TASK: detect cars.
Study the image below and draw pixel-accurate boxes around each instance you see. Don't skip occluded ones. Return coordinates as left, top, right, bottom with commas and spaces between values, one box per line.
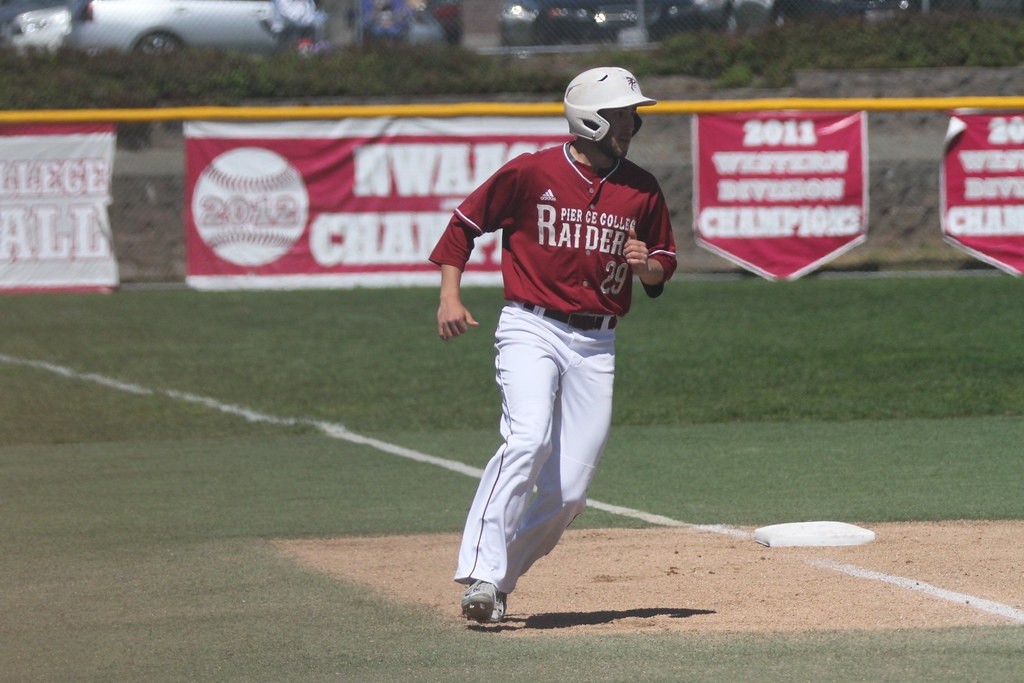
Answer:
11, 0, 319, 61
500, 0, 636, 45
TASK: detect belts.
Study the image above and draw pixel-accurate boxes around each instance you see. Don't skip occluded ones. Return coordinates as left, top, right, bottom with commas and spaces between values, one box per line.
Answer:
523, 302, 617, 331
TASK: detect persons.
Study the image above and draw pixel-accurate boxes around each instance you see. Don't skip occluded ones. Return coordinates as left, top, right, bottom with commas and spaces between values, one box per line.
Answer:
427, 66, 677, 623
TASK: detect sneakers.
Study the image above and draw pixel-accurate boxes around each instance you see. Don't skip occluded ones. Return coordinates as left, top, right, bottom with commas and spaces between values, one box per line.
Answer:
461, 580, 508, 625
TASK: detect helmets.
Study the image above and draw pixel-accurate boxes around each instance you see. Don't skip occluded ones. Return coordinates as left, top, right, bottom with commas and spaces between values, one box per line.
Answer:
564, 66, 659, 142
